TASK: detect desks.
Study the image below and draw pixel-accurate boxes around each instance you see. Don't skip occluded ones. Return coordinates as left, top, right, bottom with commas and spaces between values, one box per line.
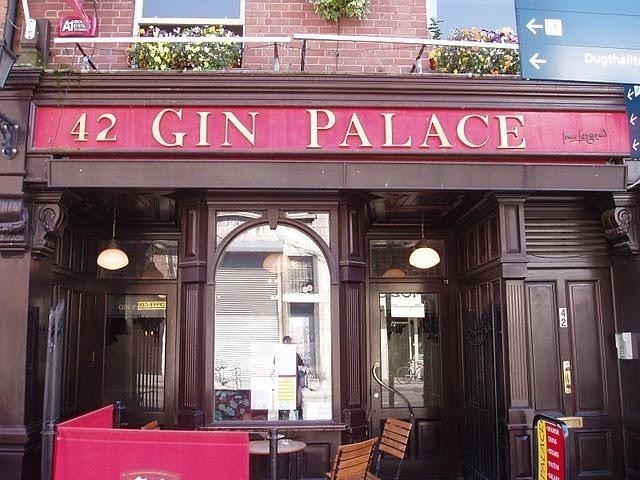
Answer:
249, 439, 307, 479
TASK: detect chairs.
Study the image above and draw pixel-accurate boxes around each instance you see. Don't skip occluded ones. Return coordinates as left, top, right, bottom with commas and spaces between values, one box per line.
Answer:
141, 420, 161, 430
296, 416, 413, 480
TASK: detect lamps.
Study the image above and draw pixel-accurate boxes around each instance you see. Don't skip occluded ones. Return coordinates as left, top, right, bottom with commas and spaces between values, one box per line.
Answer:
409, 208, 441, 269
0, 37, 20, 159
97, 201, 130, 270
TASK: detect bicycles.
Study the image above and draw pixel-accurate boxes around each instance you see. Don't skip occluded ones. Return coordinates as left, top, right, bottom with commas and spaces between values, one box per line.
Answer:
301, 352, 320, 391
397, 356, 425, 384
215, 366, 242, 390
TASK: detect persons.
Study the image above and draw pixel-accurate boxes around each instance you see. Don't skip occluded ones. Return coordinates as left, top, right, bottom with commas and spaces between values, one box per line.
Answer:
272, 335, 307, 421
